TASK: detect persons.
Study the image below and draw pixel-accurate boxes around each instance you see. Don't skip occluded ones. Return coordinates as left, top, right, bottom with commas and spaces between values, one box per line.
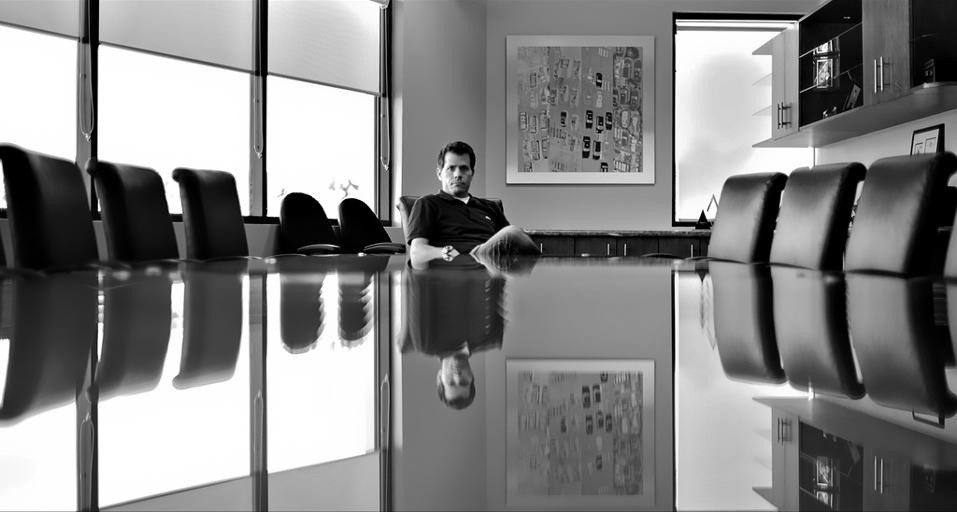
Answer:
401, 255, 508, 410
406, 137, 550, 258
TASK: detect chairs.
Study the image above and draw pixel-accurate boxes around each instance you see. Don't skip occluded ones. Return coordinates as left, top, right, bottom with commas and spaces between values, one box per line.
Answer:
275, 192, 505, 255
0, 144, 250, 271
0, 260, 242, 427
709, 152, 956, 278
710, 260, 954, 419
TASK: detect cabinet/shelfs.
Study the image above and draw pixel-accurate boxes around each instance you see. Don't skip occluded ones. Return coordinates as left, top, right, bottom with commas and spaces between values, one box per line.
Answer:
575, 236, 658, 257
527, 236, 574, 259
660, 236, 711, 259
750, 0, 956, 150
753, 395, 956, 511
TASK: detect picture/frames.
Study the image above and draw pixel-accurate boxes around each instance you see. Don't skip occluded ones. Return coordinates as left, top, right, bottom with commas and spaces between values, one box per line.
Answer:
504, 34, 657, 188
910, 123, 945, 155
502, 355, 656, 508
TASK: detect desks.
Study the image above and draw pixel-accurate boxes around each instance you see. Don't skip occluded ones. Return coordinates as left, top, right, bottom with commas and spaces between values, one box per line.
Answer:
3, 257, 956, 512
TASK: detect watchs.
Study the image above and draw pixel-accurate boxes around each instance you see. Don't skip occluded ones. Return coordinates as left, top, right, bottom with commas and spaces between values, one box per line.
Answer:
442, 245, 454, 256
443, 255, 453, 262
